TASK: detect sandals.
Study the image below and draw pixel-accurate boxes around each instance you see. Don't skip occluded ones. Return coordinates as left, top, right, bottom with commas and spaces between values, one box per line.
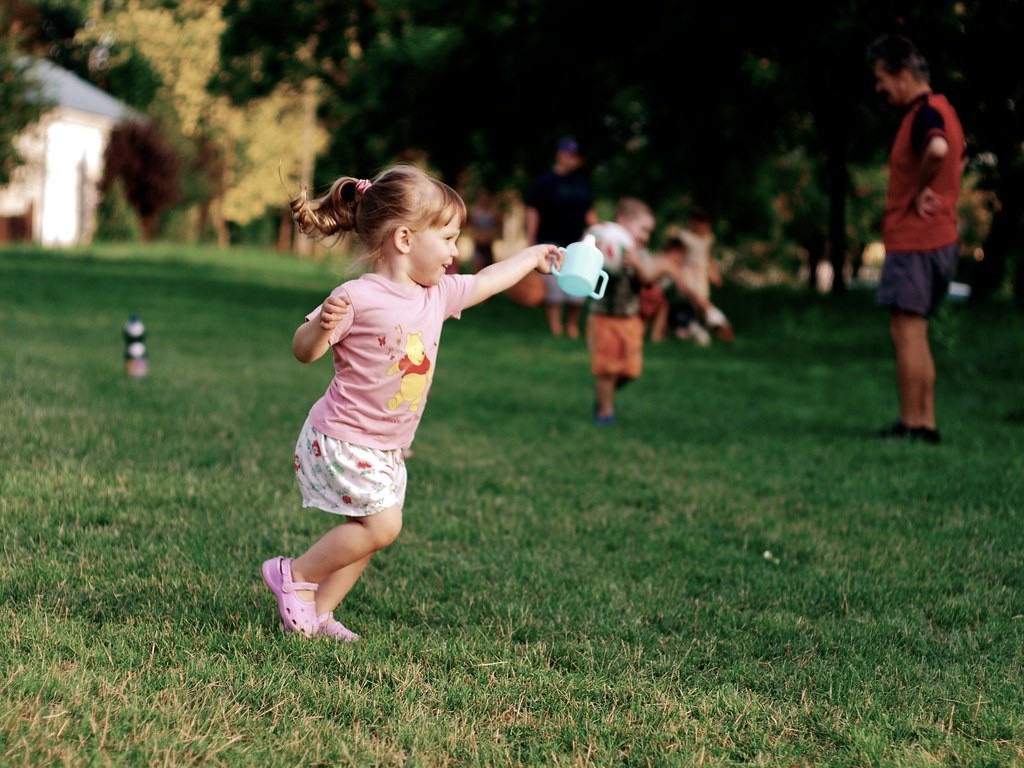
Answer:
263, 556, 320, 640
316, 611, 365, 644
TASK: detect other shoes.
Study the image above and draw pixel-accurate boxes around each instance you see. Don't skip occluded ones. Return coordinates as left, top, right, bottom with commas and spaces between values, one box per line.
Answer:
879, 420, 923, 440
918, 425, 942, 444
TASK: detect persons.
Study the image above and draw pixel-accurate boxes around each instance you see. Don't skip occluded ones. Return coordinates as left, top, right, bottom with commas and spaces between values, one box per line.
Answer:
456, 174, 738, 355
582, 197, 666, 425
257, 159, 565, 643
869, 38, 966, 447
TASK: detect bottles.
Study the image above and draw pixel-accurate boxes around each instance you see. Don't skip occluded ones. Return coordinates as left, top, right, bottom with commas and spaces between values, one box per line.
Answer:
122, 314, 149, 377
548, 233, 612, 301
